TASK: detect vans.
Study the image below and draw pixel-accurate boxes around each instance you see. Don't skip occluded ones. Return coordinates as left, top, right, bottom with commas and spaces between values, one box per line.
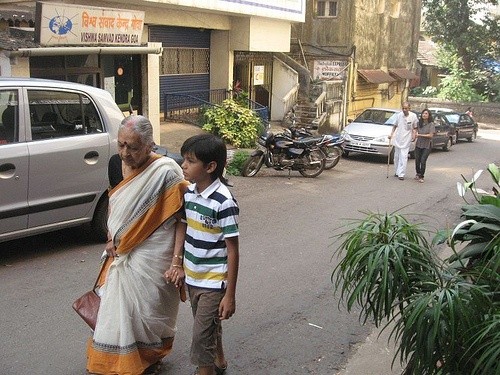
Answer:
0, 78, 126, 241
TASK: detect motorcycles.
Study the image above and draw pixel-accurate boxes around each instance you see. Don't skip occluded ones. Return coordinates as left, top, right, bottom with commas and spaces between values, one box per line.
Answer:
241, 120, 326, 179
284, 108, 346, 170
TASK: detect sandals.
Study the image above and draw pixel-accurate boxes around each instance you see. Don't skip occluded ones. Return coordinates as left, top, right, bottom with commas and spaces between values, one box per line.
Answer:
213, 361, 228, 375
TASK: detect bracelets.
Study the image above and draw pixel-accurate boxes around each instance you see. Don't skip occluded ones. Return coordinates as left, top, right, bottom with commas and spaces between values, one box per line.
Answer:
173, 253, 183, 260
169, 264, 184, 270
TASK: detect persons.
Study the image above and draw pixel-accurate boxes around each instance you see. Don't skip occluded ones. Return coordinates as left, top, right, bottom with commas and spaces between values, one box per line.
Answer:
165, 133, 240, 375
86, 115, 193, 375
414, 108, 435, 183
465, 107, 473, 118
387, 101, 419, 181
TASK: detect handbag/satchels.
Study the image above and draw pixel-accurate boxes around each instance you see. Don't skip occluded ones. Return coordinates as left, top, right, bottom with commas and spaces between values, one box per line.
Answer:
71, 289, 100, 331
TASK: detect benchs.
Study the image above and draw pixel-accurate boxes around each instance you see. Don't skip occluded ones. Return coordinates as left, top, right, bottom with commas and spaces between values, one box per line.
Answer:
37, 111, 96, 139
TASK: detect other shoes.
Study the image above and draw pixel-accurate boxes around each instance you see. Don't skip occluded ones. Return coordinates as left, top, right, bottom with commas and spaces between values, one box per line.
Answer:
415, 175, 424, 182
395, 173, 404, 180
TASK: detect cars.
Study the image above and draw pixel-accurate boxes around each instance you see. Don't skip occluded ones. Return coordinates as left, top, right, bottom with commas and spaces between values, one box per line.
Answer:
438, 111, 481, 140
411, 110, 458, 150
339, 109, 420, 160
